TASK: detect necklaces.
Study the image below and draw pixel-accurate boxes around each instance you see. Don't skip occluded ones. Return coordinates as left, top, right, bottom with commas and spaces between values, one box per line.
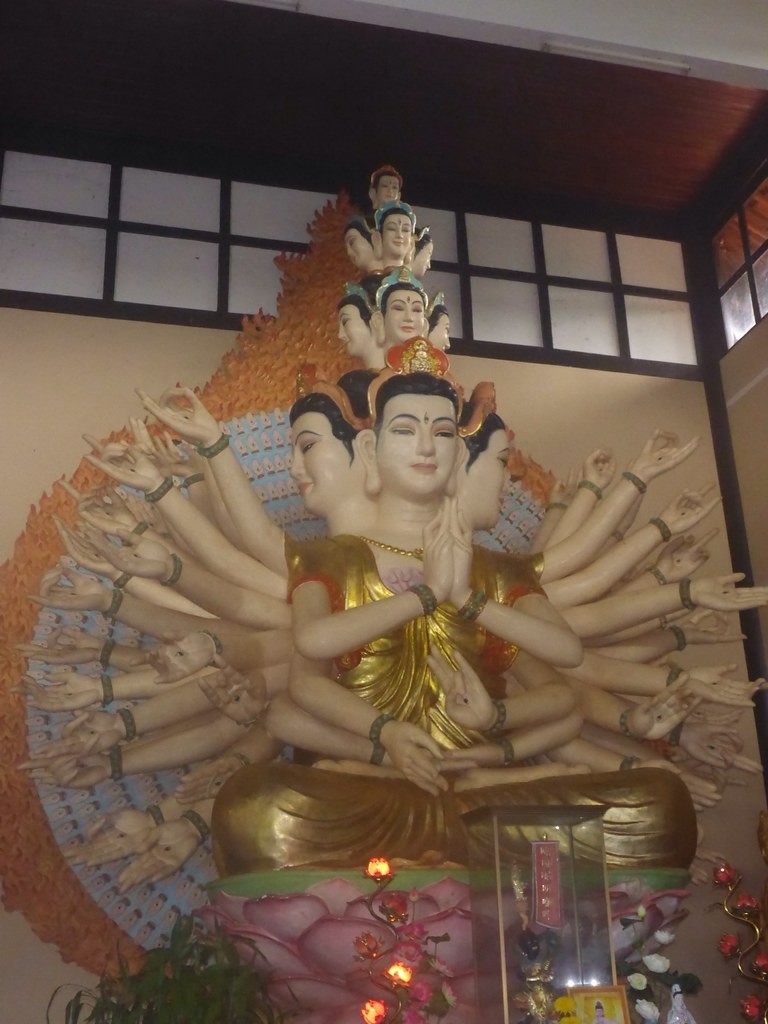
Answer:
355, 533, 426, 564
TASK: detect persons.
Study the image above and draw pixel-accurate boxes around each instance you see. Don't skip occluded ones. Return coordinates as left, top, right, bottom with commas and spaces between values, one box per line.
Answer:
9, 161, 768, 899
667, 984, 696, 1024
592, 1001, 612, 1024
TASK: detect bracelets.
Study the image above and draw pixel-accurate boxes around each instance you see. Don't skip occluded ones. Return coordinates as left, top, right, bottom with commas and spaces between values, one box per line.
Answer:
113, 572, 134, 589
644, 566, 667, 585
115, 708, 136, 742
611, 530, 624, 541
619, 708, 638, 741
493, 736, 514, 768
109, 744, 123, 781
576, 479, 603, 501
369, 746, 387, 767
665, 625, 688, 652
679, 578, 697, 611
648, 517, 671, 543
405, 582, 439, 617
618, 755, 642, 772
121, 521, 149, 546
665, 666, 684, 687
145, 804, 165, 826
102, 588, 123, 619
242, 699, 271, 728
481, 699, 508, 739
620, 472, 647, 493
196, 627, 224, 665
98, 638, 116, 672
668, 722, 684, 747
368, 713, 397, 746
659, 615, 667, 629
99, 673, 113, 706
197, 431, 230, 458
145, 476, 173, 503
231, 752, 250, 771
182, 472, 205, 489
159, 554, 183, 587
180, 809, 211, 843
545, 502, 567, 513
457, 589, 490, 627
